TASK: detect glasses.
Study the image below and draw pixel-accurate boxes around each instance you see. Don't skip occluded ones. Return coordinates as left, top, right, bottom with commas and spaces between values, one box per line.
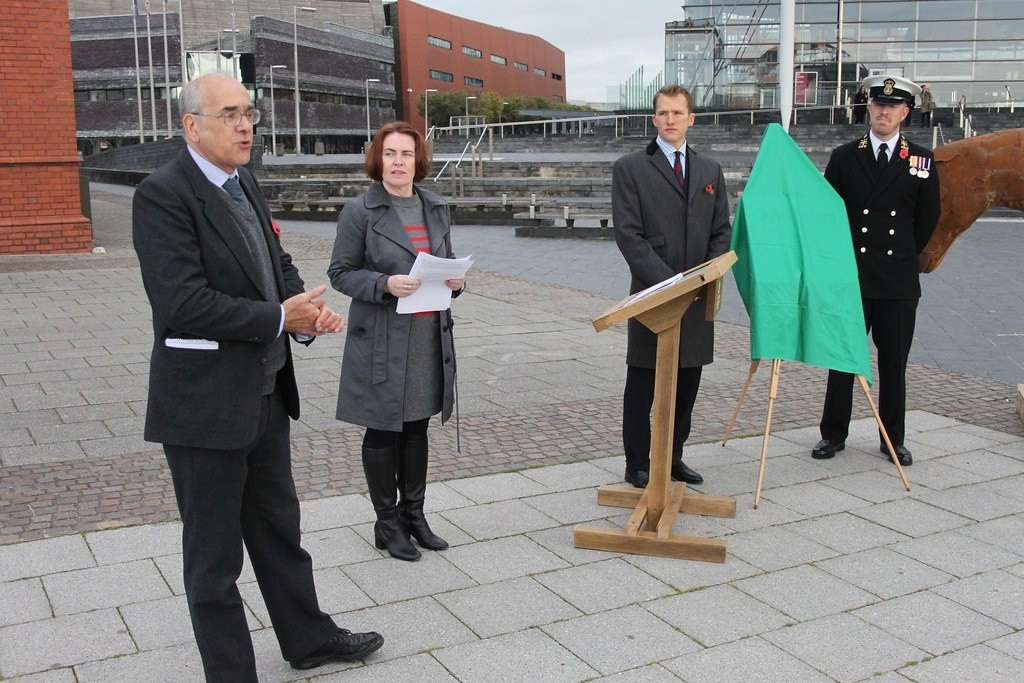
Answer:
191, 109, 263, 127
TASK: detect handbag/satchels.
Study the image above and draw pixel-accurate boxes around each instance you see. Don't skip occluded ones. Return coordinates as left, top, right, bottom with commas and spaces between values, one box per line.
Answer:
928, 102, 936, 110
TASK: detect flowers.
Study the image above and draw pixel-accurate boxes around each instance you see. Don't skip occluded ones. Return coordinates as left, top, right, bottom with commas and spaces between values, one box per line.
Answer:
272, 221, 281, 237
899, 149, 908, 160
704, 183, 715, 196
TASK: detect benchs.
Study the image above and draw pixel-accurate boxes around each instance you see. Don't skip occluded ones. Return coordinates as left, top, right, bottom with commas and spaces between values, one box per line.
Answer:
277, 192, 615, 241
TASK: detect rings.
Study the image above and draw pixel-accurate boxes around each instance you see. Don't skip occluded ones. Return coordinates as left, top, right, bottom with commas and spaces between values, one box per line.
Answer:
405, 285, 411, 290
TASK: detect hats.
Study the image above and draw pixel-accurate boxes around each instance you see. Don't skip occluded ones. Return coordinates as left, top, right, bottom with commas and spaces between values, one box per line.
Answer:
862, 75, 921, 105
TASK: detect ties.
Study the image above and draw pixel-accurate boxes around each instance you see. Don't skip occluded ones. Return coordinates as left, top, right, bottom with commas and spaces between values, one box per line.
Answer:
673, 151, 684, 187
874, 144, 889, 172
223, 177, 252, 211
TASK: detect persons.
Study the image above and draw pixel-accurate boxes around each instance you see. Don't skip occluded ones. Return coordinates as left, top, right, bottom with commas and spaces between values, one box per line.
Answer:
854, 85, 868, 124
904, 96, 915, 128
919, 85, 932, 128
611, 85, 732, 488
328, 121, 466, 560
132, 73, 384, 683
811, 74, 941, 467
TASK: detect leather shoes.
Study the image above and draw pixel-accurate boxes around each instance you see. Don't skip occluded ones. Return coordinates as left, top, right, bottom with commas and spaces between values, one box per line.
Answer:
290, 628, 384, 670
812, 439, 845, 458
880, 442, 912, 466
624, 468, 650, 489
670, 460, 703, 485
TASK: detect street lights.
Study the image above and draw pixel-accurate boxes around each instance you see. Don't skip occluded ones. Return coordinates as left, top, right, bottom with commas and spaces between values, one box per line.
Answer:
365, 78, 381, 151
466, 97, 477, 139
293, 5, 318, 155
270, 65, 288, 155
424, 88, 437, 140
216, 29, 240, 71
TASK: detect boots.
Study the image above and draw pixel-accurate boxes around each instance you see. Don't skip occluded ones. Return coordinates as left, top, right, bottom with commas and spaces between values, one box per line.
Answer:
362, 447, 421, 559
396, 439, 448, 551
